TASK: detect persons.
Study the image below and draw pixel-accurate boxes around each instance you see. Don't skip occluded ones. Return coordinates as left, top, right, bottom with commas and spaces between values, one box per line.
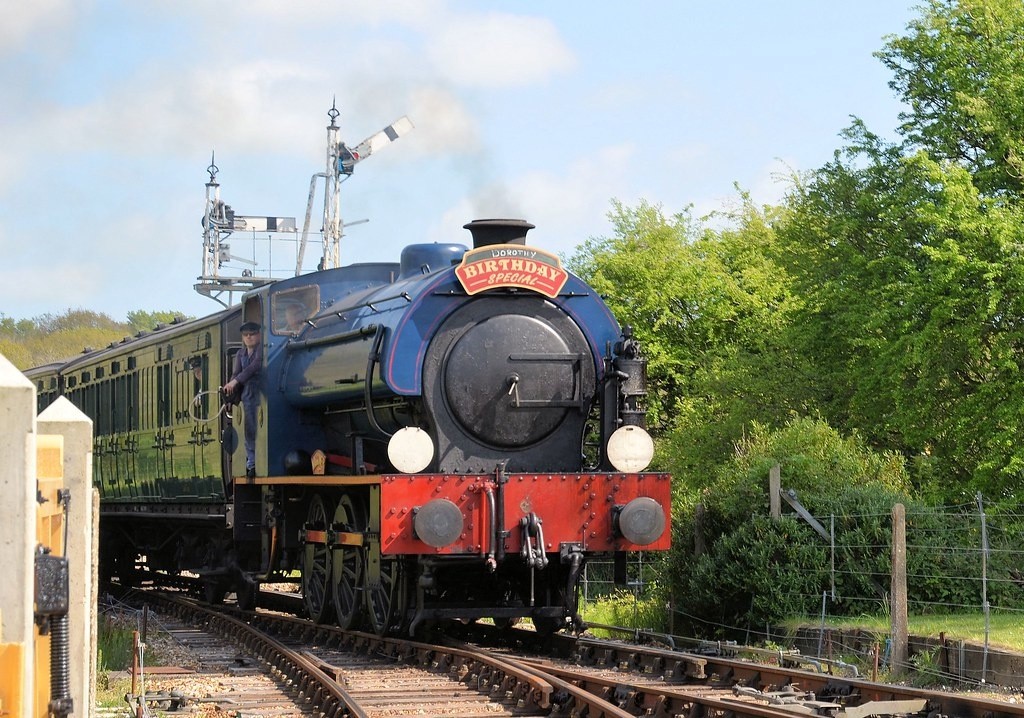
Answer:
223, 322, 261, 476
188, 356, 201, 406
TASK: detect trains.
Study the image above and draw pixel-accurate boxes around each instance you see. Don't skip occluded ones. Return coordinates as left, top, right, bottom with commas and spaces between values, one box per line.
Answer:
19, 215, 672, 635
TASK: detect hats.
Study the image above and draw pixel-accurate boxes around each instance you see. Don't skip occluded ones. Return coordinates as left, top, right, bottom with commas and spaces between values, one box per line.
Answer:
240, 322, 260, 335
189, 358, 201, 370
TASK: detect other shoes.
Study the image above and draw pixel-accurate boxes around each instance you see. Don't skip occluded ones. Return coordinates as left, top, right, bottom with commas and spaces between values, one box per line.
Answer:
247, 467, 256, 479
